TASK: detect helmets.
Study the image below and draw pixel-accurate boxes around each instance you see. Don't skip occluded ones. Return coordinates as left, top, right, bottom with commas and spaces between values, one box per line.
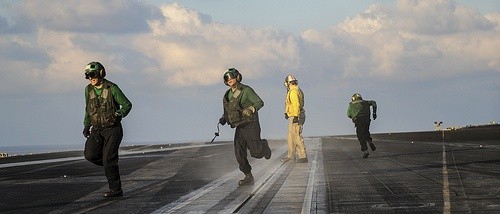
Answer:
84, 62, 104, 78
284, 76, 297, 82
352, 93, 362, 99
223, 68, 239, 82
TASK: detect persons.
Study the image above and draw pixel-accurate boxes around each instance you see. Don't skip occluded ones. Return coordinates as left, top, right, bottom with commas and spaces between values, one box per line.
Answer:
219, 67, 272, 185
347, 94, 377, 158
83, 62, 132, 197
280, 74, 308, 163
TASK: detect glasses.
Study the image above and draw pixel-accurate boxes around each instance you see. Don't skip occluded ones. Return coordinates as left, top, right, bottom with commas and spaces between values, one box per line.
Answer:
224, 73, 234, 82
86, 72, 96, 79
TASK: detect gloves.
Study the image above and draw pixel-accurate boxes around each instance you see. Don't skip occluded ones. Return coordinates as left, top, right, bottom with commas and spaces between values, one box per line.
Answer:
107, 111, 123, 126
373, 114, 377, 120
241, 106, 256, 116
284, 113, 289, 119
83, 129, 90, 137
292, 116, 299, 123
219, 114, 226, 125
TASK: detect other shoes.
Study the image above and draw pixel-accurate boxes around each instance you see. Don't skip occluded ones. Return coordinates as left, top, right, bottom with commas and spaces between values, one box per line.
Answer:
262, 139, 272, 160
297, 158, 308, 163
369, 143, 376, 151
280, 157, 295, 163
104, 189, 123, 197
363, 151, 369, 159
238, 173, 253, 186
